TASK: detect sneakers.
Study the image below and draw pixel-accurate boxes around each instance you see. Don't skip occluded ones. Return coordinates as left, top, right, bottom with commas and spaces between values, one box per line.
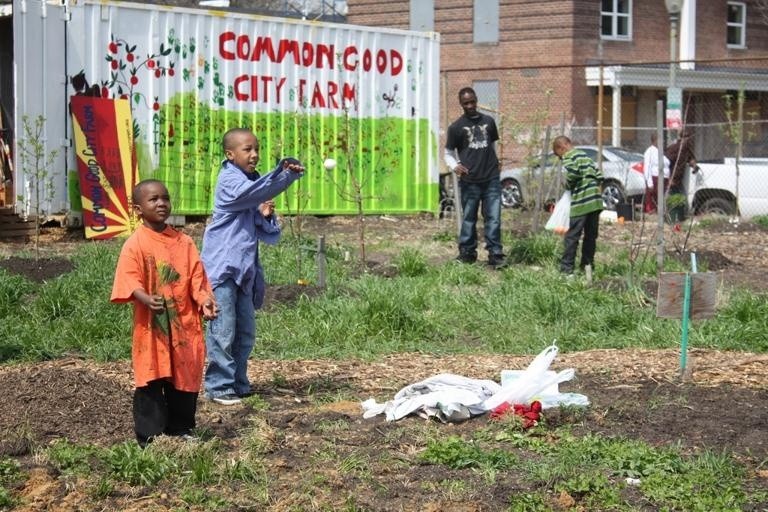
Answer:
212, 395, 242, 406
488, 254, 509, 267
456, 256, 477, 268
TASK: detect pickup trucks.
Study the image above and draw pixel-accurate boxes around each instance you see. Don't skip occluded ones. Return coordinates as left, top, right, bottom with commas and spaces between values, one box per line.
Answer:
687, 157, 767, 216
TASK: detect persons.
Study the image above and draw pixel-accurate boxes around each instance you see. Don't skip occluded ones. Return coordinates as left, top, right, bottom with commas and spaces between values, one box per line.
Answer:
552, 137, 603, 275
111, 177, 221, 451
443, 87, 508, 273
196, 127, 309, 403
642, 132, 671, 214
663, 130, 700, 223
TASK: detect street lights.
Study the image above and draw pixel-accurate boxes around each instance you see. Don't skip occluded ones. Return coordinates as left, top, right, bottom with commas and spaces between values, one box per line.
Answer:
664, 0, 684, 147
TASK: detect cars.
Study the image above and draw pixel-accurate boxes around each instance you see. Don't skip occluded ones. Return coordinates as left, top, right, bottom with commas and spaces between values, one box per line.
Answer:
499, 145, 646, 211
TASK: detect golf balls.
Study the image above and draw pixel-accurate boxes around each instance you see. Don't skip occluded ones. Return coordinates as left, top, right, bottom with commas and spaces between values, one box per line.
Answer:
325, 159, 337, 172
